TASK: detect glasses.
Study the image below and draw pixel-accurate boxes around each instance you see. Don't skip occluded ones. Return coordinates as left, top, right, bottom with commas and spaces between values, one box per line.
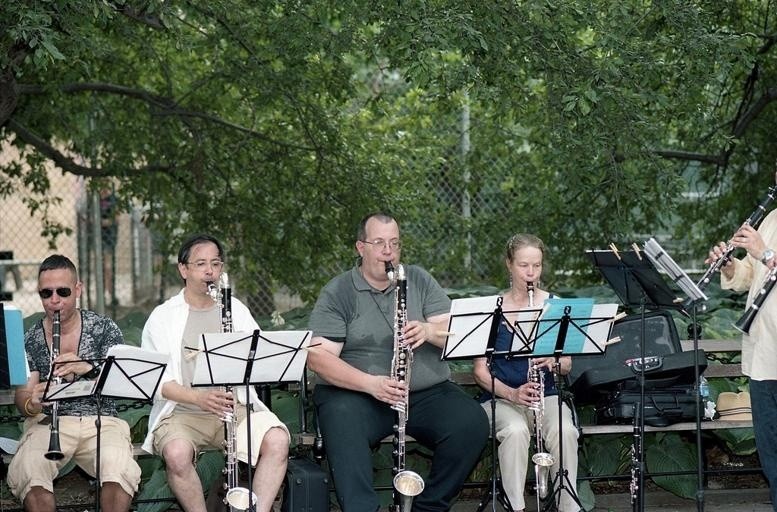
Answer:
38, 287, 75, 298
364, 240, 402, 251
188, 261, 222, 271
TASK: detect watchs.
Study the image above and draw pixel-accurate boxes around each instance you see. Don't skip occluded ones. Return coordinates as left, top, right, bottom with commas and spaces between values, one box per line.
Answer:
761, 250, 775, 266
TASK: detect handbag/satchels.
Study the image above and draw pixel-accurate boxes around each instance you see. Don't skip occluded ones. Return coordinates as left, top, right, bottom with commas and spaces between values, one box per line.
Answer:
563, 309, 708, 427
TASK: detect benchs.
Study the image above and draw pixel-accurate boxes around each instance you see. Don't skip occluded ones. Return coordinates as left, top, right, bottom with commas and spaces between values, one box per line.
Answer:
0, 386, 301, 463
291, 339, 753, 494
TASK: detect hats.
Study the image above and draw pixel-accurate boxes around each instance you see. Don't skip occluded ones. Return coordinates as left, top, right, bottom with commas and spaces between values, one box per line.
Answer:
714, 391, 752, 421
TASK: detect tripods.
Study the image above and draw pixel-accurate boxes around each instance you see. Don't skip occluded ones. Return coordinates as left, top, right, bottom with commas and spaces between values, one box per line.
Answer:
475, 362, 515, 512
541, 364, 587, 512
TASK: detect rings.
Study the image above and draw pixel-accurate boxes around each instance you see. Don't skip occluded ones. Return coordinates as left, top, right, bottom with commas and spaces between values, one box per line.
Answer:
741, 236, 747, 244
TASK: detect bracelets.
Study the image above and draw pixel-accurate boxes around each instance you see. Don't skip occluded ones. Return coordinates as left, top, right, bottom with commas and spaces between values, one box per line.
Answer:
24, 398, 42, 417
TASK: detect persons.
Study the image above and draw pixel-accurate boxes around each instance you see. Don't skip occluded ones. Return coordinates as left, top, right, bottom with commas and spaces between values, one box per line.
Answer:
306, 214, 488, 512
142, 238, 292, 511
1, 254, 142, 511
472, 233, 585, 512
703, 207, 777, 512
